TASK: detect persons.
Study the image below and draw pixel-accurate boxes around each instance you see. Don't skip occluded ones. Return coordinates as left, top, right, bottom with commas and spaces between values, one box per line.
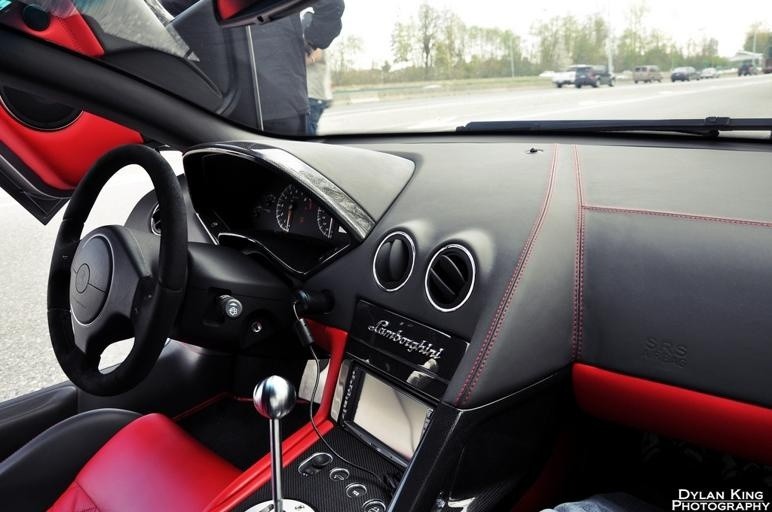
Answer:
248, 0, 346, 140
300, 12, 337, 138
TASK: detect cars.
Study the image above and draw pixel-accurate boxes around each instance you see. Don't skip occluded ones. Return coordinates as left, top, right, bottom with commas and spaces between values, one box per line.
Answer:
539, 64, 772, 88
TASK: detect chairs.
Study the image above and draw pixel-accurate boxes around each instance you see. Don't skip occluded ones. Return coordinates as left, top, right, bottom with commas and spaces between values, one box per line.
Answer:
1, 406, 245, 511
